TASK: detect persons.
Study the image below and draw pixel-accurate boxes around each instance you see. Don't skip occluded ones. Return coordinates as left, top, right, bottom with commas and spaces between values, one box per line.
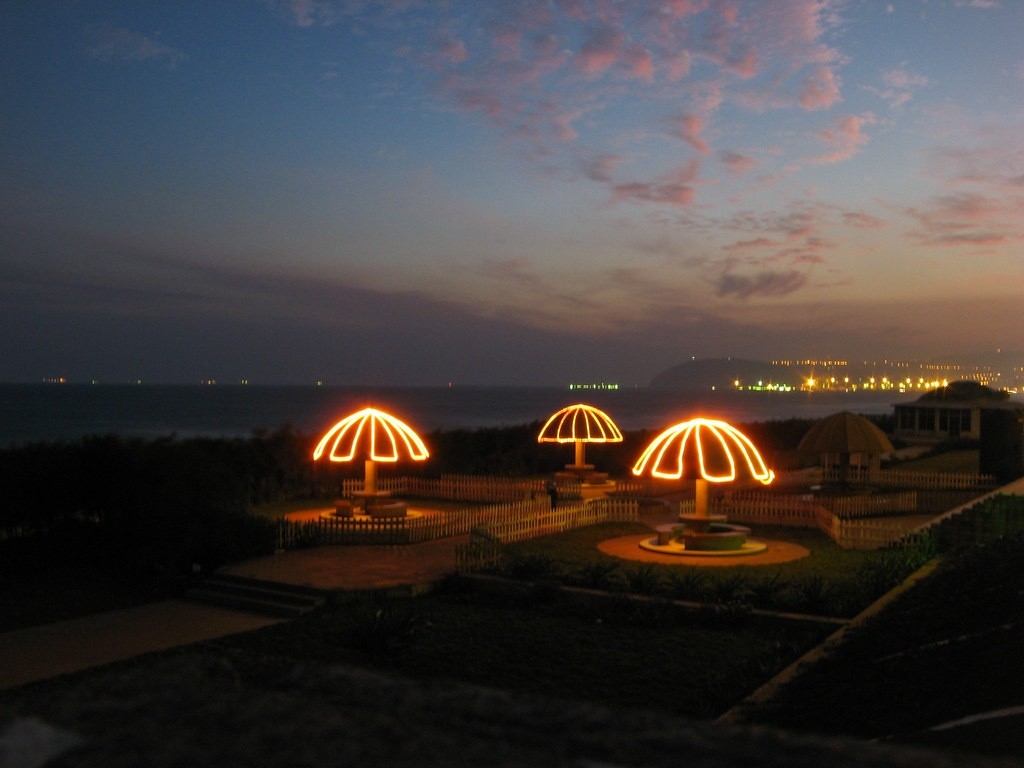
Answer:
549, 479, 558, 512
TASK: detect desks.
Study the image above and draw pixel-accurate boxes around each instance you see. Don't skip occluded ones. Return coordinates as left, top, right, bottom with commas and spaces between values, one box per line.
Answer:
829, 480, 864, 495
565, 464, 595, 482
352, 490, 391, 515
679, 514, 727, 531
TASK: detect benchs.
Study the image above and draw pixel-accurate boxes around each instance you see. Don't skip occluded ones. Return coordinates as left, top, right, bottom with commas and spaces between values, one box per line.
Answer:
810, 484, 879, 497
681, 532, 747, 550
333, 500, 351, 517
555, 471, 578, 484
368, 499, 406, 518
587, 472, 608, 484
655, 523, 751, 545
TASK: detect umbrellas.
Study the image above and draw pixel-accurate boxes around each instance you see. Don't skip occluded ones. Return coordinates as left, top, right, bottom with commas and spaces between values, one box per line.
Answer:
537, 404, 624, 476
632, 418, 776, 532
796, 411, 896, 494
312, 408, 430, 517
